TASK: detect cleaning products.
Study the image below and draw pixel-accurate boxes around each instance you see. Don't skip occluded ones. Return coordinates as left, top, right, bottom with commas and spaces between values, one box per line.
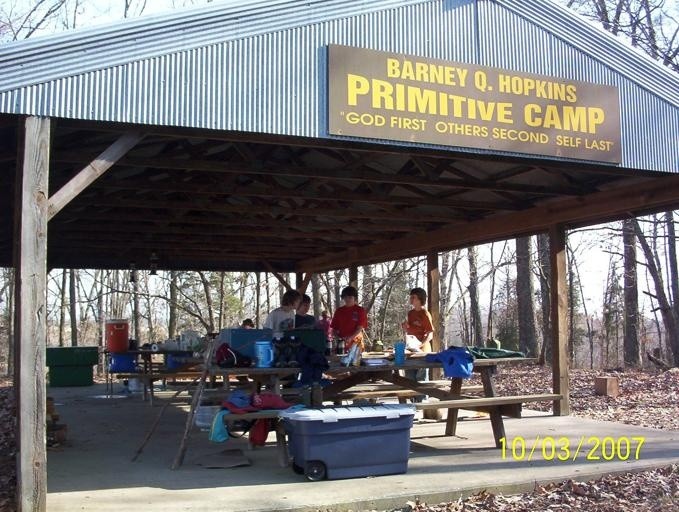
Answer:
395, 342, 405, 365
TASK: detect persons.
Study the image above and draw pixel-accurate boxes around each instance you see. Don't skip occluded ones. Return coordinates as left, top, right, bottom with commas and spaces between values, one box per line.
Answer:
402, 288, 435, 420
296, 292, 317, 329
238, 318, 254, 329
329, 287, 368, 352
315, 311, 334, 357
263, 290, 303, 341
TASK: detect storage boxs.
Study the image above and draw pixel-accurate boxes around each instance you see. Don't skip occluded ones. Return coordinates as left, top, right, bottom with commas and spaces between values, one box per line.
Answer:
277, 403, 415, 479
220, 328, 327, 364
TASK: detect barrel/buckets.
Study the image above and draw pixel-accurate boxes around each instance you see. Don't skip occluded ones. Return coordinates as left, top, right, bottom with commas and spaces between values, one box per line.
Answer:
106, 319, 128, 353
168, 351, 192, 369
254, 342, 274, 368
110, 339, 137, 373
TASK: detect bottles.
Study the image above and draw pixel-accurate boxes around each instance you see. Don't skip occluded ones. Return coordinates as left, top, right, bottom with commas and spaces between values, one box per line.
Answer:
394, 339, 406, 366
336, 338, 346, 355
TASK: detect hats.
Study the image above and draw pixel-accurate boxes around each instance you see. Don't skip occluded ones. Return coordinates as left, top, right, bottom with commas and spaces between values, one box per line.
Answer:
243, 319, 254, 327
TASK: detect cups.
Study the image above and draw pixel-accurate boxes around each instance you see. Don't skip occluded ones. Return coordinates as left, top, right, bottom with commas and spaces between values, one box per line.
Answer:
253, 340, 275, 369
